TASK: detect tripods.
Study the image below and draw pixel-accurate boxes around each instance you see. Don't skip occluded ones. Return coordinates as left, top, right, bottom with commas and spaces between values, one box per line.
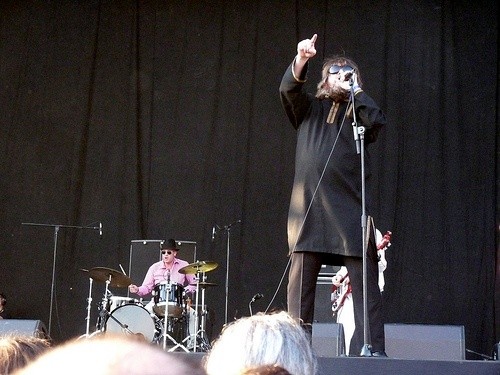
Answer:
153, 270, 211, 354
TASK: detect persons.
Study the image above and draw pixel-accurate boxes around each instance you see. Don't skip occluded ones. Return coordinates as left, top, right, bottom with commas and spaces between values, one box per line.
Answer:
128, 238, 199, 353
332, 228, 387, 357
0, 311, 319, 375
279, 34, 388, 356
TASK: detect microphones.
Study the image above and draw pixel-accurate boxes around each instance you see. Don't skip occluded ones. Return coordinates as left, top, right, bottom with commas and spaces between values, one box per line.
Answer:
212, 225, 216, 241
338, 69, 354, 81
98, 221, 103, 239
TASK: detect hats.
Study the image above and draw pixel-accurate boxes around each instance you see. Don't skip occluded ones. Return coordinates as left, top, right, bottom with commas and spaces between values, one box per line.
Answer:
160, 238, 179, 251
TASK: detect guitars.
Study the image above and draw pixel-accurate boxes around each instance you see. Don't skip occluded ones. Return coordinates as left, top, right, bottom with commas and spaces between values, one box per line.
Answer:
330, 230, 392, 312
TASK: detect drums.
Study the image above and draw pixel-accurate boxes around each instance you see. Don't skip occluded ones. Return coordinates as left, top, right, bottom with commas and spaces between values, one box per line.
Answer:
109, 296, 134, 312
105, 303, 157, 343
152, 281, 185, 318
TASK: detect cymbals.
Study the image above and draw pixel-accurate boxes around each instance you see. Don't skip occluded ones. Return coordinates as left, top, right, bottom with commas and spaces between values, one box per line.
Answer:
89, 267, 133, 288
178, 261, 218, 275
188, 283, 216, 287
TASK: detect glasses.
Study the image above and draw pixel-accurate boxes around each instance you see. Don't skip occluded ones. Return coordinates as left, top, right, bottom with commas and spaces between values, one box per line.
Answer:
161, 250, 172, 255
328, 64, 354, 74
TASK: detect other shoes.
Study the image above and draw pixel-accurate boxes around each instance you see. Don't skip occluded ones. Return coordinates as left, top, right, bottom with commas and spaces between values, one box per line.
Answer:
364, 350, 389, 358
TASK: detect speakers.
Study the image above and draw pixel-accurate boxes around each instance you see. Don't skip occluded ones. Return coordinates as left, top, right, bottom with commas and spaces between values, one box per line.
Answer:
1, 319, 48, 339
313, 282, 341, 324
381, 322, 468, 361
309, 322, 346, 357
